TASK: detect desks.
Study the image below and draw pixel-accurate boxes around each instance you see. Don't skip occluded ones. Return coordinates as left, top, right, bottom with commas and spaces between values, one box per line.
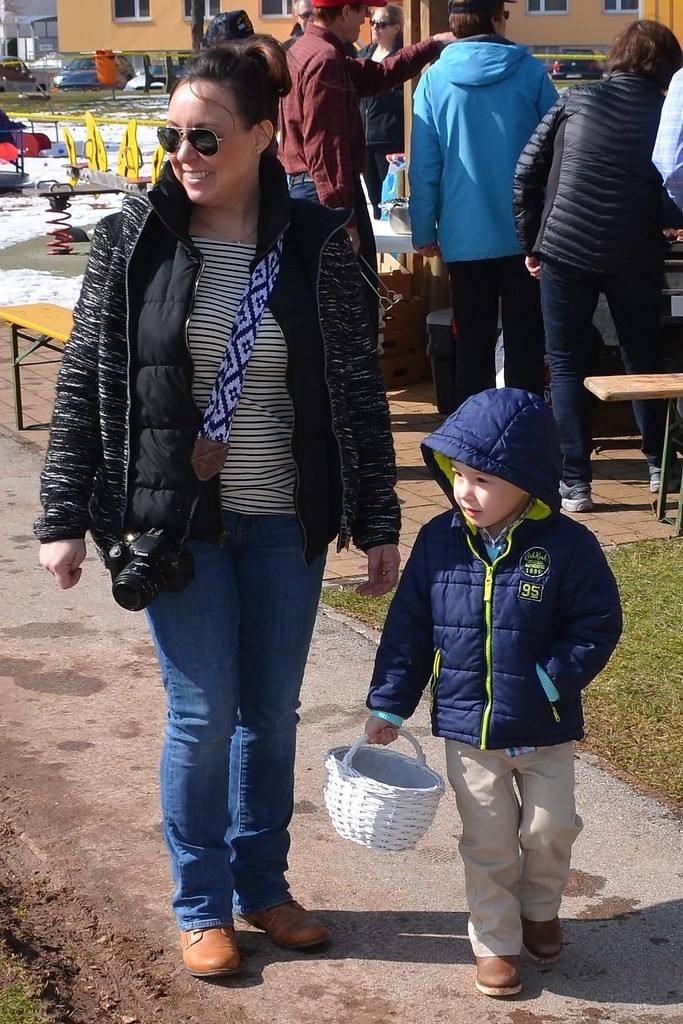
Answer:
372, 219, 418, 252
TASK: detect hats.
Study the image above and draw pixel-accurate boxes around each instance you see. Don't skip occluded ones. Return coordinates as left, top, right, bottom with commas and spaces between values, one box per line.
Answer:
446, 0, 519, 14
310, 0, 387, 7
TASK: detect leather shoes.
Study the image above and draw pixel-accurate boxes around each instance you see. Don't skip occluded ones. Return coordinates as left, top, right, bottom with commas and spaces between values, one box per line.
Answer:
473, 955, 523, 994
181, 916, 242, 976
232, 894, 330, 947
519, 914, 563, 964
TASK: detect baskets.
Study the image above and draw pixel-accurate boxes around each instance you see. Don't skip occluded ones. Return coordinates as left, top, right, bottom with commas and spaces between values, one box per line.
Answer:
323, 727, 447, 850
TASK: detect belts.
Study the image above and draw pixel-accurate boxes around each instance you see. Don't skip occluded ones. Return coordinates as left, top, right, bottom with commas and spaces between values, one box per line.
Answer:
288, 172, 316, 185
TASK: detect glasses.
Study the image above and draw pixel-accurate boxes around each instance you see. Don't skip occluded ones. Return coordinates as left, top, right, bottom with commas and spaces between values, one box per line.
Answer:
296, 12, 312, 21
157, 124, 255, 159
369, 18, 397, 30
493, 11, 510, 20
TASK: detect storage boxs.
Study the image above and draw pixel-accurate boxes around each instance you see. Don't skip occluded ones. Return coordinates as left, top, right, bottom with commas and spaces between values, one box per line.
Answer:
376, 252, 422, 386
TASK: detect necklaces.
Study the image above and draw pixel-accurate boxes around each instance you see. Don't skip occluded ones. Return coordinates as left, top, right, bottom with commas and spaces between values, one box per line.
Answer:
193, 212, 258, 244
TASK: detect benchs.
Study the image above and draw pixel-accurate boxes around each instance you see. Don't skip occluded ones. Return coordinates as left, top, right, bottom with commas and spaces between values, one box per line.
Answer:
0, 303, 76, 430
583, 373, 683, 536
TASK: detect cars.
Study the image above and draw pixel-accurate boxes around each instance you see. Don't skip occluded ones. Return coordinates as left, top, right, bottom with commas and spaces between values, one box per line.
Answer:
123, 65, 185, 93
53, 55, 135, 92
552, 48, 603, 80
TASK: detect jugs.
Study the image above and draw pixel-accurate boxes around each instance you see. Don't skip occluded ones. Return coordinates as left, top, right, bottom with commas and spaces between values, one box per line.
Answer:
378, 197, 412, 235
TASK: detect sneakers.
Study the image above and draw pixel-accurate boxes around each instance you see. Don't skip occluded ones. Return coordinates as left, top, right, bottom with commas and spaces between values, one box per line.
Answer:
648, 462, 682, 493
559, 480, 594, 512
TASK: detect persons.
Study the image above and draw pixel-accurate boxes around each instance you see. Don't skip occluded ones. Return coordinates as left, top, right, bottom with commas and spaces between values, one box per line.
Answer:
357, 0, 404, 222
367, 383, 622, 1001
650, 59, 683, 210
512, 19, 683, 511
408, 0, 561, 415
33, 44, 403, 978
274, 0, 457, 355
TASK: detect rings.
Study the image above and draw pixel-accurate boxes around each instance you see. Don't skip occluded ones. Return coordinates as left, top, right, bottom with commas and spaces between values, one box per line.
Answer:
381, 572, 387, 575
531, 273, 533, 276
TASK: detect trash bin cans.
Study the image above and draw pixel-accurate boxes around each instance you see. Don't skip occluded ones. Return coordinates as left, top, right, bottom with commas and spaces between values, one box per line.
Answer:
425, 307, 457, 415
93, 49, 117, 86
